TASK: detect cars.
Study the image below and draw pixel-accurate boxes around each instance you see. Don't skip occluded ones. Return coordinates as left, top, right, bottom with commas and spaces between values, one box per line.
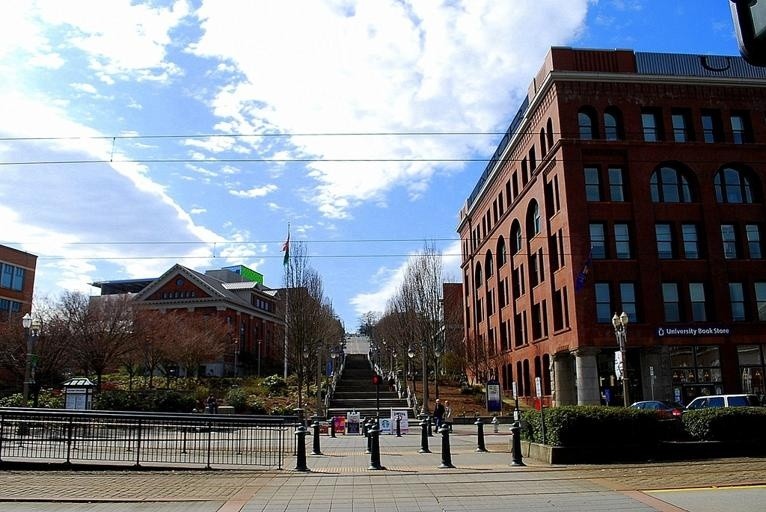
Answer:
630, 394, 760, 432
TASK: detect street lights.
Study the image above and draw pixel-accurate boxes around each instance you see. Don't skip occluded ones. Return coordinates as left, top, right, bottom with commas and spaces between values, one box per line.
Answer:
611, 311, 630, 408
303, 346, 336, 416
369, 339, 407, 398
233, 337, 239, 378
408, 342, 440, 415
334, 336, 351, 384
18, 313, 42, 436
257, 339, 263, 377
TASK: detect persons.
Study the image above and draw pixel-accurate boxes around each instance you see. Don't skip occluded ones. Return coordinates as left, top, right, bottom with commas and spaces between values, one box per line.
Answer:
433, 399, 453, 433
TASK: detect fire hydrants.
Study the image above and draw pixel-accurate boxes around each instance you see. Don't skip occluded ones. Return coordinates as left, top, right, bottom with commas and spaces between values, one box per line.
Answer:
491, 417, 500, 433
